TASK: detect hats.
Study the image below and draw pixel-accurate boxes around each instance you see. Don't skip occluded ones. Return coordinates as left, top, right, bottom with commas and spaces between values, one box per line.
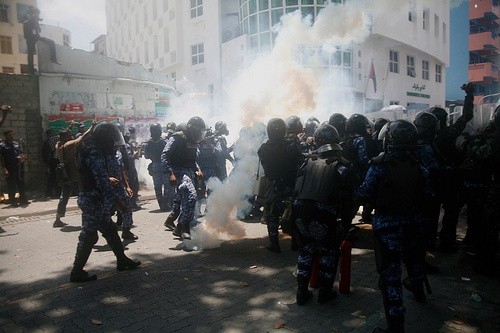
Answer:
76, 121, 86, 127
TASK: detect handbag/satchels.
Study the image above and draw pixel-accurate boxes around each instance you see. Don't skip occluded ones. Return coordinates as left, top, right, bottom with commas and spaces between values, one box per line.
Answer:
54, 163, 68, 184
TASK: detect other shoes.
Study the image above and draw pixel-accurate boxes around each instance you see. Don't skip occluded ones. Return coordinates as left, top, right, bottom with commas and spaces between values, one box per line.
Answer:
173, 224, 192, 239
403, 277, 426, 302
116, 251, 141, 270
292, 236, 299, 251
296, 288, 313, 304
318, 288, 337, 304
70, 269, 97, 282
164, 218, 177, 230
266, 243, 282, 253
372, 327, 388, 333
53, 221, 66, 227
122, 231, 138, 240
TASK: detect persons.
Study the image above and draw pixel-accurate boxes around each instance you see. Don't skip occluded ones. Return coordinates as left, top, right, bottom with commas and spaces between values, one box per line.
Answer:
42, 117, 269, 241
69, 121, 142, 281
261, 82, 500, 333
23, 8, 62, 71
0, 130, 31, 206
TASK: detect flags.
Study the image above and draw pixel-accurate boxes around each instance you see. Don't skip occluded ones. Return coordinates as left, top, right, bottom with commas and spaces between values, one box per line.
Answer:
369, 62, 376, 93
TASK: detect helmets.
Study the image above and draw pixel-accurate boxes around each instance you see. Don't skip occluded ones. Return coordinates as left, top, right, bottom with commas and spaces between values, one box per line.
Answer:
267, 105, 449, 159
150, 117, 229, 144
112, 122, 136, 142
93, 121, 121, 154
